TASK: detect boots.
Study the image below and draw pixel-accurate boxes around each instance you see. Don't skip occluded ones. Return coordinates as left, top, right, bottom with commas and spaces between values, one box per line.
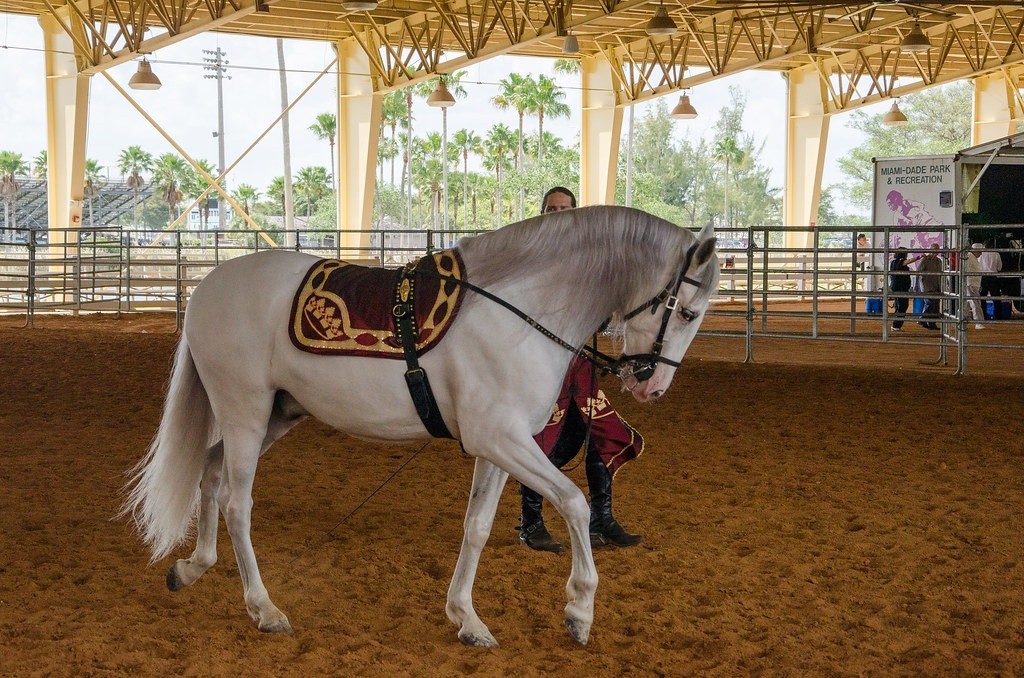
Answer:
584, 459, 643, 546
519, 483, 563, 552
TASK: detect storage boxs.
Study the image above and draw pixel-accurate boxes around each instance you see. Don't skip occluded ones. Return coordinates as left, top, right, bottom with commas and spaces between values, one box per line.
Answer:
984, 300, 1013, 319
865, 297, 882, 314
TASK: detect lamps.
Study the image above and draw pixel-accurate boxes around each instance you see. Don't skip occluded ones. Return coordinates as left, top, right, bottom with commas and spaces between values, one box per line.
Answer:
671, 88, 698, 120
426, 73, 456, 107
901, 15, 930, 53
128, 51, 161, 90
644, 0, 678, 35
341, 0, 378, 11
881, 100, 908, 125
562, 28, 579, 54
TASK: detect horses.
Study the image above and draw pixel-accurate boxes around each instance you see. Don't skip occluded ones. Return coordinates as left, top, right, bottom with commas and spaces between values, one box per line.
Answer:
109, 205, 723, 648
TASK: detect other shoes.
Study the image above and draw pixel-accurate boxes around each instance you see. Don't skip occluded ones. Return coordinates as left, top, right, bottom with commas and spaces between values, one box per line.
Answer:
975, 323, 986, 329
891, 326, 905, 332
916, 320, 940, 330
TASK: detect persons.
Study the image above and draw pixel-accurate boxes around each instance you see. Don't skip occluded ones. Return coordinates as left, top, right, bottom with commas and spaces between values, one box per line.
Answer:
956, 244, 988, 331
518, 186, 645, 553
856, 234, 871, 267
890, 246, 925, 331
977, 240, 1007, 320
915, 243, 942, 330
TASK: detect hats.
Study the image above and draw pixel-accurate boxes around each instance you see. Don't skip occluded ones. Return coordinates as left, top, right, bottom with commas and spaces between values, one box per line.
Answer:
972, 244, 983, 249
